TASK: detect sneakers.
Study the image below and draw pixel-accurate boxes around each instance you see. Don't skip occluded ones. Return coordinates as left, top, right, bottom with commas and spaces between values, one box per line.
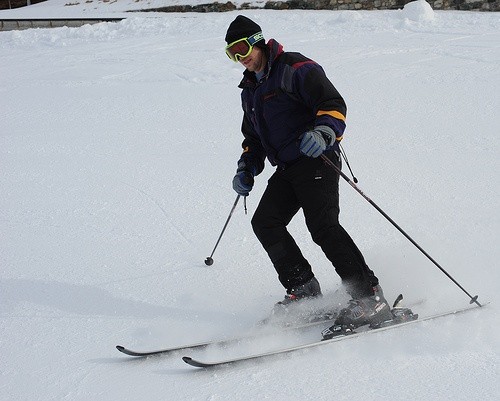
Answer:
275, 278, 322, 309
334, 284, 390, 323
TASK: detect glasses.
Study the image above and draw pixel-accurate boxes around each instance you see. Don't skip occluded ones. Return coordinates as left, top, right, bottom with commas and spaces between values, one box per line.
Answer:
225, 31, 264, 63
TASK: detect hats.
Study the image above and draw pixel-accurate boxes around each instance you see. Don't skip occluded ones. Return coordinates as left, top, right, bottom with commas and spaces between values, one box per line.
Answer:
225, 14, 265, 47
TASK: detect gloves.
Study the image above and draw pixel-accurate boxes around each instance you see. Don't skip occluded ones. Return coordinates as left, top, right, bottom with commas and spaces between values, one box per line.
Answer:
233, 162, 256, 196
299, 126, 336, 159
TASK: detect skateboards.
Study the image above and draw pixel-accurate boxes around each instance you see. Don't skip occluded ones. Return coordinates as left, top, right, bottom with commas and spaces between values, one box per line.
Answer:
116, 293, 488, 368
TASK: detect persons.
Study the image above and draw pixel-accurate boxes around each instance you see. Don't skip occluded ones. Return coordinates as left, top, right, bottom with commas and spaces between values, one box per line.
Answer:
224, 14, 393, 327
